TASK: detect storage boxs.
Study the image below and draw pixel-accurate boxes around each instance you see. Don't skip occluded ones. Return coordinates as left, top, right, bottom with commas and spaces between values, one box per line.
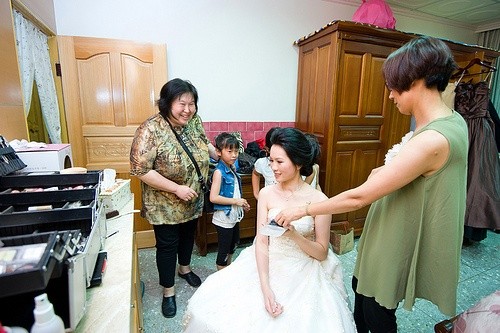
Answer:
0, 167, 109, 333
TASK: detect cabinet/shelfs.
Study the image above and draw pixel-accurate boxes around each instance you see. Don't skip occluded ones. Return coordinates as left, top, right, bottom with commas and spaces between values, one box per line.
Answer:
78, 192, 145, 333
195, 173, 265, 257
293, 19, 500, 238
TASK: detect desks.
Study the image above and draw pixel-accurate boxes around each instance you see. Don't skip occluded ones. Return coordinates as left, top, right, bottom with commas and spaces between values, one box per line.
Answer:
12, 143, 74, 171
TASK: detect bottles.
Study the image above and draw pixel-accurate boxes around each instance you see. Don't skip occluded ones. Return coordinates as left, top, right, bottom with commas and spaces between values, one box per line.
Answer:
31, 293, 66, 333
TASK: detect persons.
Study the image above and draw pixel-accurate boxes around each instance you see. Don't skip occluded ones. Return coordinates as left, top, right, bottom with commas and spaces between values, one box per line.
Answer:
274, 36, 468, 333
191, 128, 357, 333
252, 128, 323, 201
130, 78, 221, 318
209, 133, 250, 270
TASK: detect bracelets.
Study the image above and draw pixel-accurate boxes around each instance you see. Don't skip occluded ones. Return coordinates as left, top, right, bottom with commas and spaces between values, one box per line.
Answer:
306, 202, 311, 216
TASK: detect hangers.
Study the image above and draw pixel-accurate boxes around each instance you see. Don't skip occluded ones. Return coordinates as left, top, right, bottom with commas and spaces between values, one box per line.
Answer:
449, 51, 497, 79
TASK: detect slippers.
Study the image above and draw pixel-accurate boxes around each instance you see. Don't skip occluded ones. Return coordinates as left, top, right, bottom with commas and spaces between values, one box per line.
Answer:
162, 294, 176, 318
178, 271, 202, 287
